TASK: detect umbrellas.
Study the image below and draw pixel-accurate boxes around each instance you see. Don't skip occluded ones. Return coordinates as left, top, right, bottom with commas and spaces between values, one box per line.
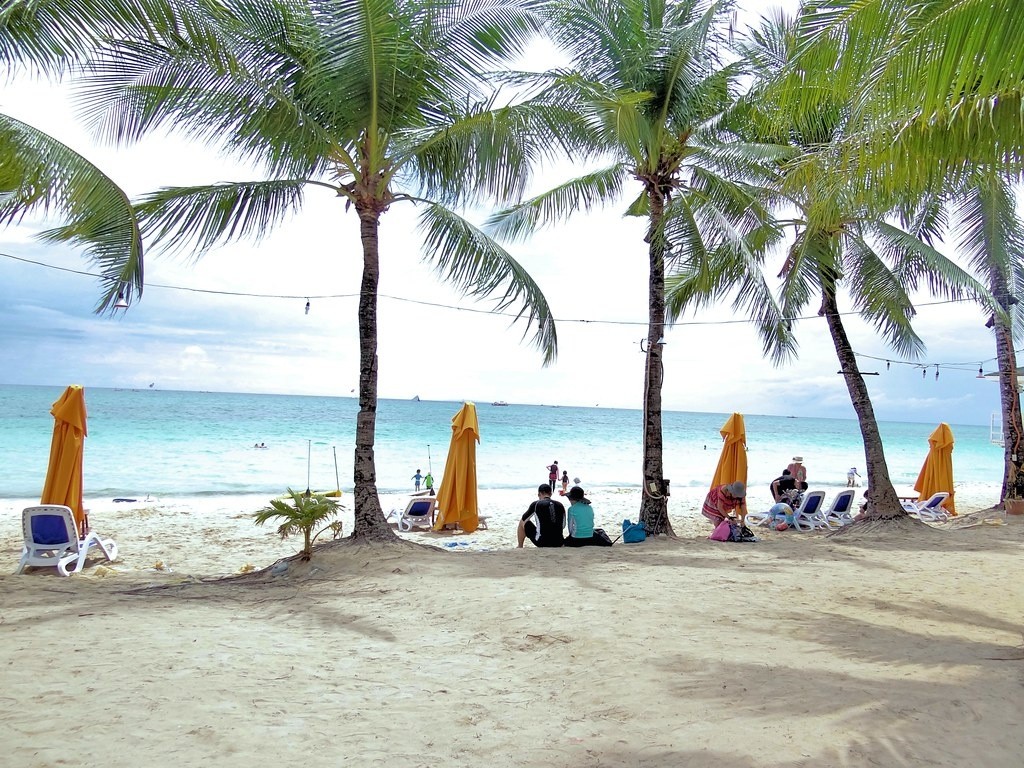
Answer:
709, 412, 748, 517
41, 384, 87, 538
431, 402, 480, 533
913, 422, 958, 516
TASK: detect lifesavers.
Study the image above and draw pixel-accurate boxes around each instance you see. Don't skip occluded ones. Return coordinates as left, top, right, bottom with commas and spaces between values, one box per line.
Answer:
768, 502, 793, 531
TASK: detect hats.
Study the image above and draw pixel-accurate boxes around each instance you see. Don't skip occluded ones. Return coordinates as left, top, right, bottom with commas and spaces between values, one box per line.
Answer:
851, 467, 856, 469
564, 487, 590, 503
792, 456, 803, 463
727, 482, 746, 497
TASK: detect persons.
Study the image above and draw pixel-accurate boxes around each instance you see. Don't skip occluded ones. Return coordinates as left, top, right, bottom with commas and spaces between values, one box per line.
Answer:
702, 481, 745, 529
546, 461, 559, 493
847, 467, 861, 487
516, 484, 566, 548
558, 471, 569, 492
770, 456, 808, 515
261, 443, 265, 446
255, 444, 258, 447
563, 486, 594, 547
422, 471, 434, 490
411, 469, 424, 492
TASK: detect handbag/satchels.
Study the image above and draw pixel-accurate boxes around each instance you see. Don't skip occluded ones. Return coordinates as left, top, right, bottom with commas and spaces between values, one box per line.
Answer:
621, 520, 647, 543
708, 520, 756, 542
594, 528, 612, 545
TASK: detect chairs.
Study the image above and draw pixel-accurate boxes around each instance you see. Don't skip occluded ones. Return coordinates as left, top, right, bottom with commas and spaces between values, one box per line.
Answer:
13, 505, 117, 576
384, 497, 436, 532
744, 493, 950, 533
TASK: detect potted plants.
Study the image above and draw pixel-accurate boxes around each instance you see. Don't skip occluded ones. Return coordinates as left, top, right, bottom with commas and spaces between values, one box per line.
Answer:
1003, 488, 1024, 515
